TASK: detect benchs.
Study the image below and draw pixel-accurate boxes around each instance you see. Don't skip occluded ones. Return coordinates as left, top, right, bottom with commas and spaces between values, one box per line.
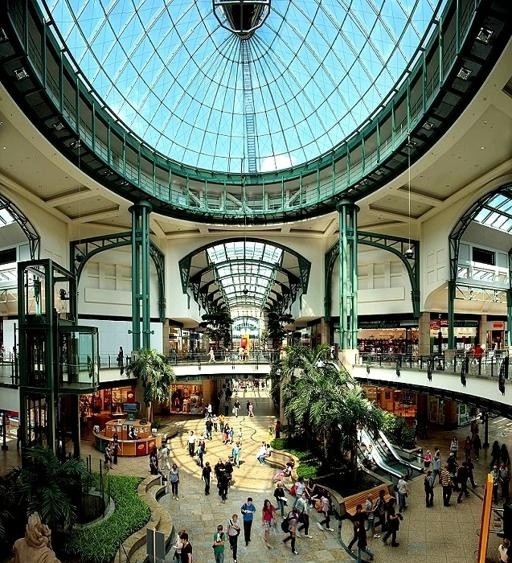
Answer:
343, 483, 394, 521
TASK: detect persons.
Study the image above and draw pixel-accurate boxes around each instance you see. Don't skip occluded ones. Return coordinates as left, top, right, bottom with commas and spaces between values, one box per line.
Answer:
16, 425, 21, 450
0, 413, 4, 436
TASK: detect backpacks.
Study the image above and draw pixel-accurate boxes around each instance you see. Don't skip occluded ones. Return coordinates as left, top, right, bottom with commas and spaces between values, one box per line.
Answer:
280, 511, 296, 533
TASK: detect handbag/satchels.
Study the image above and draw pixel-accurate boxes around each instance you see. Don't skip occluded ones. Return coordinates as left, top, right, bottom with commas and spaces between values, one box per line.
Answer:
315, 500, 322, 511
373, 507, 384, 516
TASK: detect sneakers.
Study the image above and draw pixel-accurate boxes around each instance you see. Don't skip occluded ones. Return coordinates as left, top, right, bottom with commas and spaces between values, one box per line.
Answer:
293, 550, 298, 555
305, 535, 312, 538
382, 538, 388, 545
392, 543, 399, 547
374, 534, 381, 537
325, 527, 334, 531
317, 522, 324, 531
